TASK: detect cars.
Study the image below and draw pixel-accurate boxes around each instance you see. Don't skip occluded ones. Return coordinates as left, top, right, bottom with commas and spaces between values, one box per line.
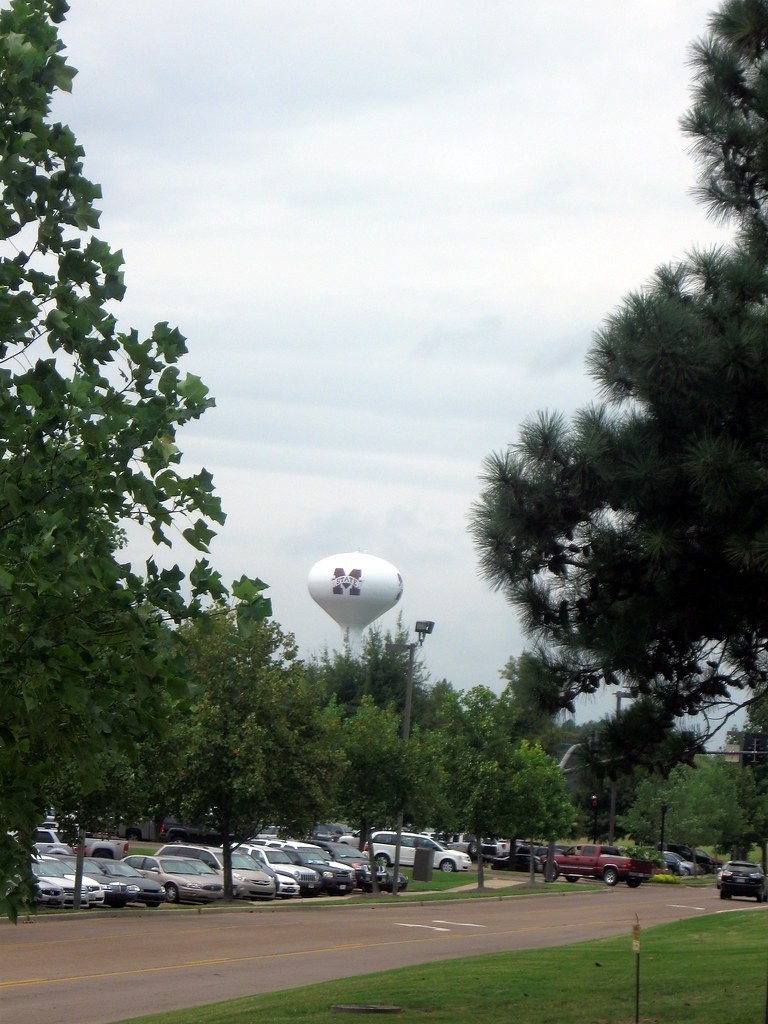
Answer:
33, 808, 129, 860
35, 878, 65, 909
659, 844, 723, 876
220, 824, 409, 899
31, 859, 90, 909
159, 817, 234, 848
420, 832, 562, 872
31, 854, 105, 904
41, 853, 138, 908
116, 815, 178, 841
84, 857, 167, 907
122, 855, 223, 904
717, 861, 732, 889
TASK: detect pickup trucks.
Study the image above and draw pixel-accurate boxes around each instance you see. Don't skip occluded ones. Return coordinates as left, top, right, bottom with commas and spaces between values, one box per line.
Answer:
540, 844, 653, 888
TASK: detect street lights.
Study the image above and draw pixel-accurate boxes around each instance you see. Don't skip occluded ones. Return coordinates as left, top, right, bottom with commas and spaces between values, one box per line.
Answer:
393, 621, 435, 897
609, 691, 638, 846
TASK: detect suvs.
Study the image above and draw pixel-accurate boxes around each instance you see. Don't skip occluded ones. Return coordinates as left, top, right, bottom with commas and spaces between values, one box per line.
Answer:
363, 830, 473, 874
154, 843, 276, 899
720, 860, 768, 903
